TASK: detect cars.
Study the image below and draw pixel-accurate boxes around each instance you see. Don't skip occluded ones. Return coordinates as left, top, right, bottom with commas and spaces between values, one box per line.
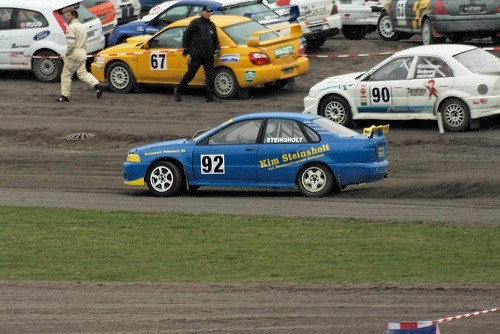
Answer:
122, 112, 390, 198
377, 0, 500, 46
302, 44, 500, 132
108, 0, 307, 55
90, 15, 311, 100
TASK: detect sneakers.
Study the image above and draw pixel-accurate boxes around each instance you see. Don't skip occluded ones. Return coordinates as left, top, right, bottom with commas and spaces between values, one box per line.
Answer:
97, 84, 104, 98
56, 96, 70, 102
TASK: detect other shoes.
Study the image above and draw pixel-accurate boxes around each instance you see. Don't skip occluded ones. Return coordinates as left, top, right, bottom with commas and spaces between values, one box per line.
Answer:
174, 88, 182, 101
207, 97, 223, 103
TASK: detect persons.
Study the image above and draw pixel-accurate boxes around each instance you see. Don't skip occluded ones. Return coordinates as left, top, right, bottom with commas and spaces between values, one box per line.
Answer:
54, 10, 103, 102
174, 4, 221, 102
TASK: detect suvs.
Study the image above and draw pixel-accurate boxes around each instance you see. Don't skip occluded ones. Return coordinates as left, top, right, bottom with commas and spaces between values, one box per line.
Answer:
0, 0, 105, 83
81, 0, 119, 41
339, 0, 385, 40
262, 0, 343, 51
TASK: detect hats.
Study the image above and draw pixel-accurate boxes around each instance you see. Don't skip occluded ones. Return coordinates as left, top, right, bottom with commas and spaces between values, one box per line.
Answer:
202, 5, 214, 12
